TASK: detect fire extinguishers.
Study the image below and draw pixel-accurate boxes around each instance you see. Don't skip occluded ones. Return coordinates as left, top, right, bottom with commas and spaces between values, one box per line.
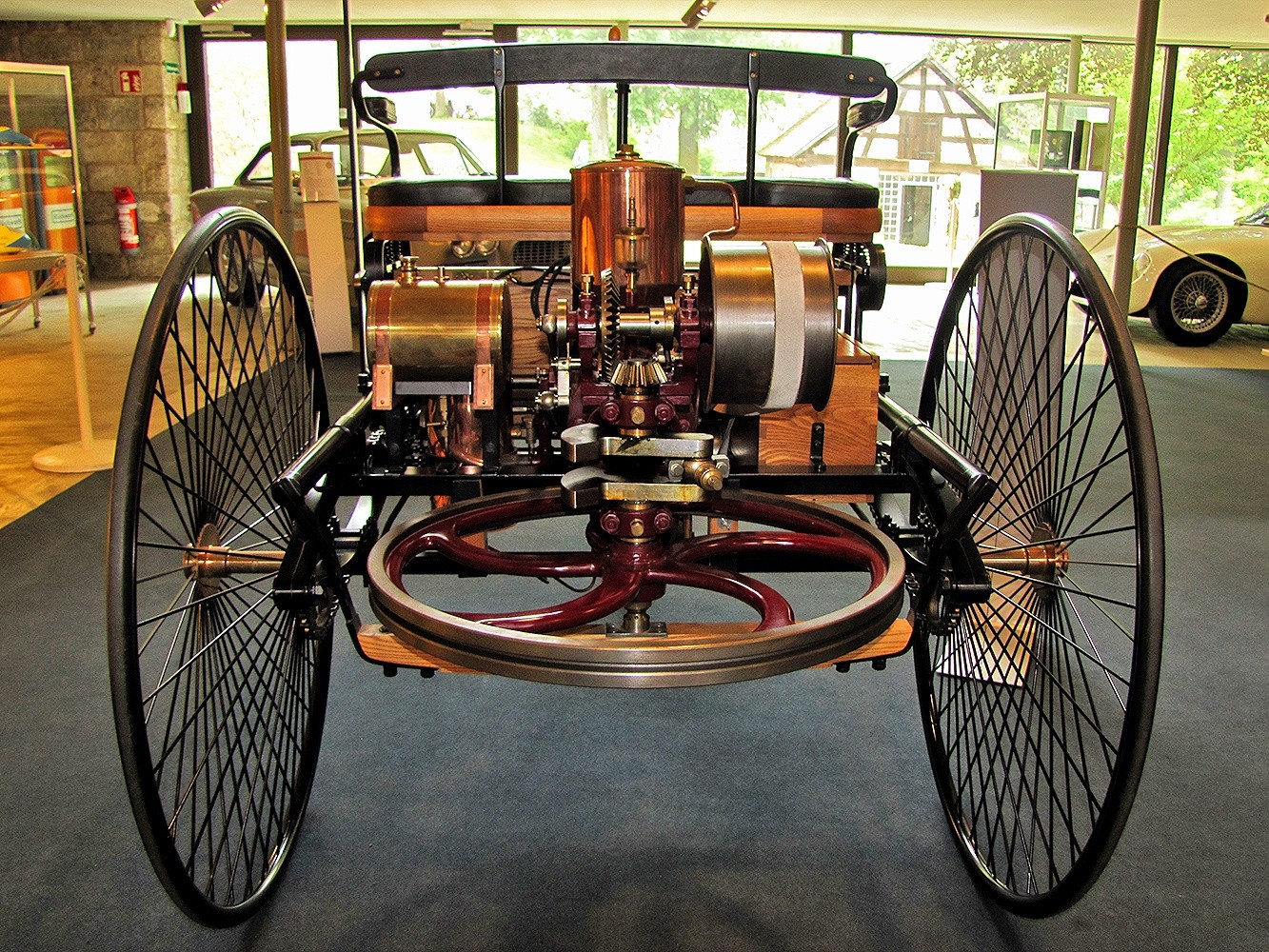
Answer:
115, 186, 142, 258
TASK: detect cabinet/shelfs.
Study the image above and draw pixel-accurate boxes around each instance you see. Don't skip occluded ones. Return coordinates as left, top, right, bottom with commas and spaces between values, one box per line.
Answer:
996, 92, 1116, 232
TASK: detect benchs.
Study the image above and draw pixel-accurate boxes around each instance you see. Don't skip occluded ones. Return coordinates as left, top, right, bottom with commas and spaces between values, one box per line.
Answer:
352, 46, 894, 236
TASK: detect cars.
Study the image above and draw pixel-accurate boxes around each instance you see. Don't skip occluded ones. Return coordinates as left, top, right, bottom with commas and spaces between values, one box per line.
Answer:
1067, 201, 1268, 348
190, 128, 497, 308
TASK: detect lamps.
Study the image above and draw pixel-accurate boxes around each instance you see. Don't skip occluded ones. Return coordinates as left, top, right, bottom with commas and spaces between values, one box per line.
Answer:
195, 0, 229, 17
682, 0, 718, 29
178, 91, 192, 114
